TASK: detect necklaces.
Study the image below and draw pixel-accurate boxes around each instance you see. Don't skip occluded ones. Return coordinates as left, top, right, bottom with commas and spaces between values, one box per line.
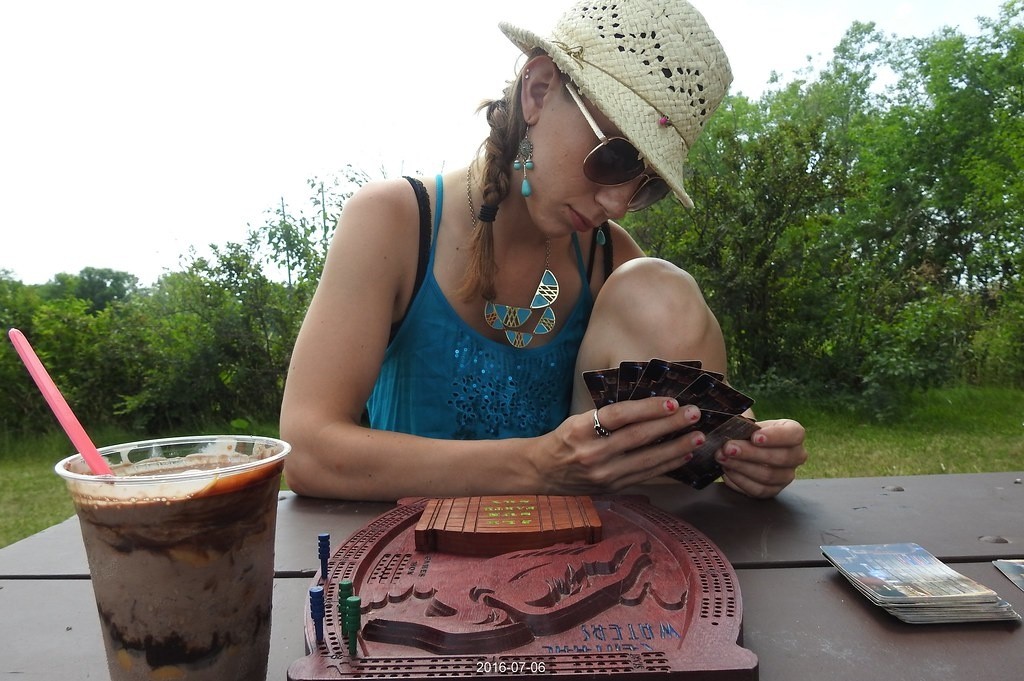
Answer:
466, 158, 560, 349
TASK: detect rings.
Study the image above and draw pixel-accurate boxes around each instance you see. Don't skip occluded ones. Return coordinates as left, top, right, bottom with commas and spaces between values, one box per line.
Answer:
593, 410, 611, 438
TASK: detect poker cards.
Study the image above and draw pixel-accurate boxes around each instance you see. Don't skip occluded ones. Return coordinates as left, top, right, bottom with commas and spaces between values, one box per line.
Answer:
584, 356, 759, 492
819, 542, 1024, 623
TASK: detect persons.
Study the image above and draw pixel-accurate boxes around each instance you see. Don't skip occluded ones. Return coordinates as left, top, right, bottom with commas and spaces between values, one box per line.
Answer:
278, 0, 808, 501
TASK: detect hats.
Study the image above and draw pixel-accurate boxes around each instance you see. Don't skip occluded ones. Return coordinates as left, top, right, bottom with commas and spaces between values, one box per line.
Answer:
497, 0, 735, 209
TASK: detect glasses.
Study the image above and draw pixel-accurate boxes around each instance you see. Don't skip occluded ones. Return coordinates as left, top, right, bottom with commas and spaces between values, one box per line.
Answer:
565, 82, 672, 212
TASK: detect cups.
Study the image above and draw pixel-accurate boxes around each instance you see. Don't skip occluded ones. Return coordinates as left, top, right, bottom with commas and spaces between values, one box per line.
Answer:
54, 433, 293, 681
